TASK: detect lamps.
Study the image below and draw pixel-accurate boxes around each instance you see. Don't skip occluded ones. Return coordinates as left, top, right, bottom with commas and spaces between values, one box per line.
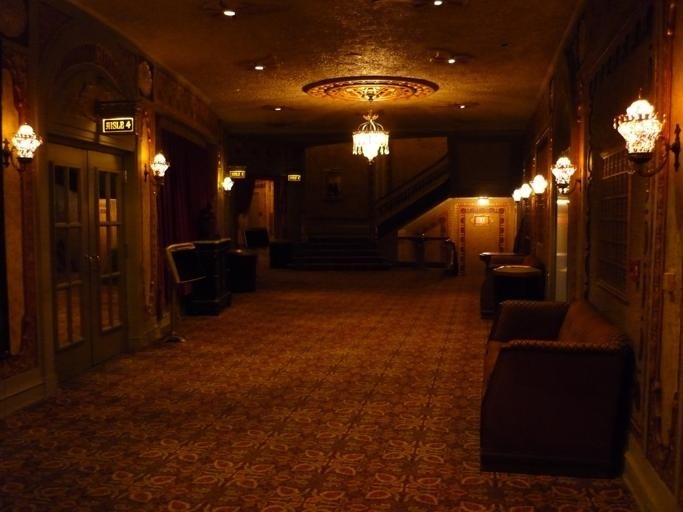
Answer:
0, 118, 44, 168
218, 176, 234, 196
350, 92, 391, 165
610, 96, 682, 181
510, 155, 581, 206
143, 148, 171, 186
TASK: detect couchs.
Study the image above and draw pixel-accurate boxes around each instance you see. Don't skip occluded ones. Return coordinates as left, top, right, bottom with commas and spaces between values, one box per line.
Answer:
476, 295, 635, 473
